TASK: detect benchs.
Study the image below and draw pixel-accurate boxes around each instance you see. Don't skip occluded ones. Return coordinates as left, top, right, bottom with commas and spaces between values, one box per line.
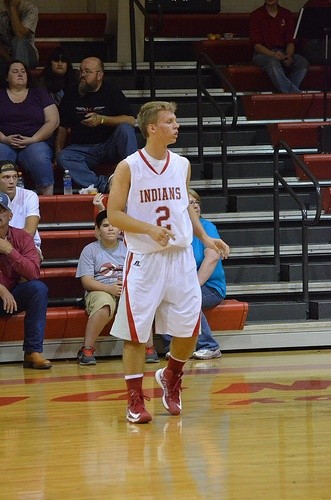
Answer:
0, 11, 331, 341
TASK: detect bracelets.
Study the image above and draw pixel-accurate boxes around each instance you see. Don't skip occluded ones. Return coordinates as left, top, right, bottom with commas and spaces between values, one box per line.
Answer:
100, 115, 104, 125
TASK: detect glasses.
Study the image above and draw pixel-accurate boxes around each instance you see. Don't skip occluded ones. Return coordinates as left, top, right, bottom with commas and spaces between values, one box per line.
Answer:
78, 70, 100, 75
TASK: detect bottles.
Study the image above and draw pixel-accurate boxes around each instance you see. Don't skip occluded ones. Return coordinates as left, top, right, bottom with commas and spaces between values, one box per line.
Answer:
17, 172, 24, 188
63, 170, 72, 195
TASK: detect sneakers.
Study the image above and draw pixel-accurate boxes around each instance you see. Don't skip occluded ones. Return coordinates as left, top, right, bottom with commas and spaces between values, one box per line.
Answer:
74, 346, 97, 365
126, 422, 153, 436
125, 389, 153, 423
146, 346, 160, 364
155, 366, 183, 415
193, 348, 222, 360
165, 350, 170, 359
158, 416, 182, 462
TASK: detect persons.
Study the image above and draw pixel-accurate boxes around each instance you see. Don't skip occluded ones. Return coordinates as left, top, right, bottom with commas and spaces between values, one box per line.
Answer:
106, 102, 230, 423
74, 210, 159, 363
157, 189, 227, 360
0, 160, 52, 370
0, 0, 39, 70
93, 171, 127, 246
0, 51, 140, 195
248, 0, 308, 93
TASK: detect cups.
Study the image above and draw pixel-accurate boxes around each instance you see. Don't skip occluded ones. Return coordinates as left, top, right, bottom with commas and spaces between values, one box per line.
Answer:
224, 33, 233, 40
88, 188, 98, 194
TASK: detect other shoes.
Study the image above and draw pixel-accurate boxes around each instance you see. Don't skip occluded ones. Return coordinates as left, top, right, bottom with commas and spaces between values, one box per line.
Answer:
23, 351, 52, 368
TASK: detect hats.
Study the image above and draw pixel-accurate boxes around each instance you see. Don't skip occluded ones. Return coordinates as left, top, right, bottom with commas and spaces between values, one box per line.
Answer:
0, 160, 18, 174
0, 192, 12, 211
